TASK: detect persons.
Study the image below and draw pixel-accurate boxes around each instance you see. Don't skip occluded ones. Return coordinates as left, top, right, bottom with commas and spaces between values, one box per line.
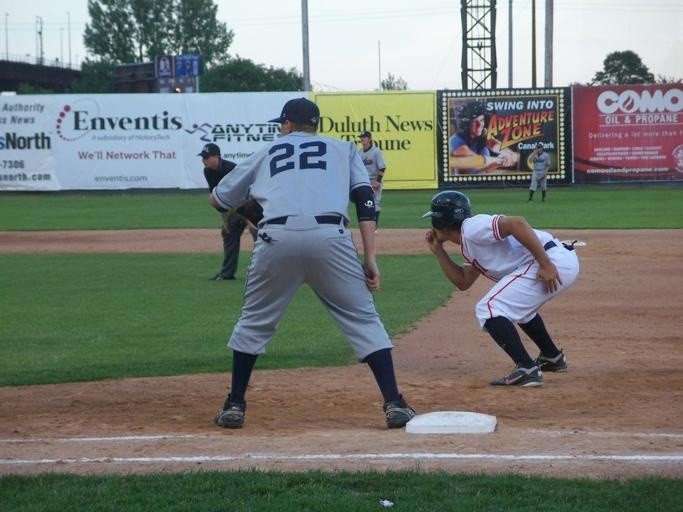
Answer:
356, 131, 386, 235
422, 189, 580, 388
528, 143, 550, 201
447, 102, 519, 175
197, 142, 263, 282
206, 96, 417, 428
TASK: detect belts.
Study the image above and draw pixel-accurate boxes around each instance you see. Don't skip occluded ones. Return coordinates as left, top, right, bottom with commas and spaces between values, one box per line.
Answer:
544, 241, 556, 251
267, 216, 341, 224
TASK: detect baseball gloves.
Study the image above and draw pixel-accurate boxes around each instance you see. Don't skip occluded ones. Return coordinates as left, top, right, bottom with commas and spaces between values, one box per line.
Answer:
237, 193, 262, 228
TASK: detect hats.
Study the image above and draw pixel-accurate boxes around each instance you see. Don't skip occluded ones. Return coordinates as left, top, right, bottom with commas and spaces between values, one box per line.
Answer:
357, 131, 371, 137
197, 144, 220, 159
267, 98, 319, 126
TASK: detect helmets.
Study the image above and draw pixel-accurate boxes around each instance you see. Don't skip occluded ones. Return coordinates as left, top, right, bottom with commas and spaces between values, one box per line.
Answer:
421, 191, 471, 218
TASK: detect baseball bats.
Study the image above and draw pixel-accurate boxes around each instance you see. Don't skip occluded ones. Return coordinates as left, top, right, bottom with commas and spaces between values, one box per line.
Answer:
452, 154, 506, 170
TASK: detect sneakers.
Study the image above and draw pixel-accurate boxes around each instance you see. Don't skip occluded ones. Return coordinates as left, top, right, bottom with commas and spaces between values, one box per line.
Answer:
489, 359, 545, 387
383, 394, 416, 428
211, 274, 236, 280
215, 394, 246, 428
537, 348, 568, 371
